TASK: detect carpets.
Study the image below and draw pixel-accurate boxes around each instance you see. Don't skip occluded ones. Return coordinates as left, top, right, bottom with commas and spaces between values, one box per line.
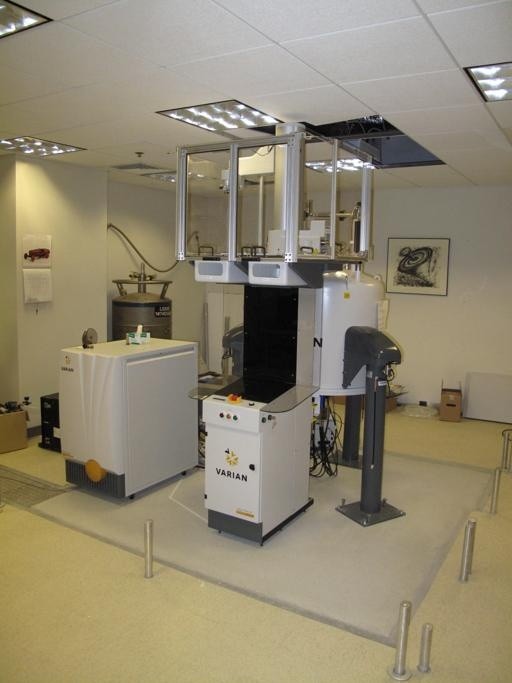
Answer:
28, 440, 495, 648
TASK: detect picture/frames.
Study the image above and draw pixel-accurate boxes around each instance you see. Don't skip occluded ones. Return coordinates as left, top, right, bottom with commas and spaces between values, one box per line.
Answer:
387, 237, 450, 296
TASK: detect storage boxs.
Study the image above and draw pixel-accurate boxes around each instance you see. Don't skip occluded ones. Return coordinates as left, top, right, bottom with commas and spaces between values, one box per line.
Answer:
364, 391, 408, 412
0, 409, 31, 453
439, 379, 462, 422
